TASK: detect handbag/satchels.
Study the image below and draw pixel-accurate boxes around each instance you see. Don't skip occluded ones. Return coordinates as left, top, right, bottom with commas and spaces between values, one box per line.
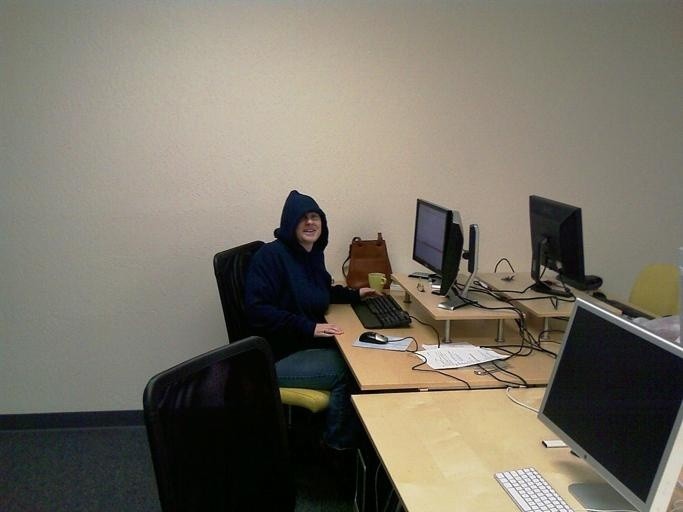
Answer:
347, 240, 393, 289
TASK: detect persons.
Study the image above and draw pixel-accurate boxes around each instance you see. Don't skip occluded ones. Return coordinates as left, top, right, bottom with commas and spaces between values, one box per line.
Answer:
244, 190, 384, 451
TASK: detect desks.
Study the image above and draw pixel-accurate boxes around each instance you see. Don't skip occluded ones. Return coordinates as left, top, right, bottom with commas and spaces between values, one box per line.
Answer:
345, 386, 647, 511
315, 267, 613, 388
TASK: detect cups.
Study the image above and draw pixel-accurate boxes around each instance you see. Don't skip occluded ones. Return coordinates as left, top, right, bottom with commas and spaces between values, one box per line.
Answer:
368, 272, 387, 292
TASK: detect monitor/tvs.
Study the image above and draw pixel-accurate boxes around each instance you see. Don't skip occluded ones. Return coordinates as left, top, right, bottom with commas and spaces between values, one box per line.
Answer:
408, 198, 452, 279
439, 210, 477, 311
529, 195, 584, 297
537, 298, 683, 512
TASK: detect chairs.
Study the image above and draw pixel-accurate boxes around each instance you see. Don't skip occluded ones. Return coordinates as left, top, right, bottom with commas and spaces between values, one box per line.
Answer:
624, 261, 680, 317
140, 334, 302, 512
210, 240, 343, 429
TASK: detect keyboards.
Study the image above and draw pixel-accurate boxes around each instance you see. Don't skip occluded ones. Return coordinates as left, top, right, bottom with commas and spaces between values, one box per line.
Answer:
604, 300, 655, 320
351, 295, 412, 329
494, 466, 575, 512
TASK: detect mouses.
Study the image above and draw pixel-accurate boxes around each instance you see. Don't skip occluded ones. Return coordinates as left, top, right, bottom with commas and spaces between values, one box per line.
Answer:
359, 331, 388, 344
593, 291, 606, 299
